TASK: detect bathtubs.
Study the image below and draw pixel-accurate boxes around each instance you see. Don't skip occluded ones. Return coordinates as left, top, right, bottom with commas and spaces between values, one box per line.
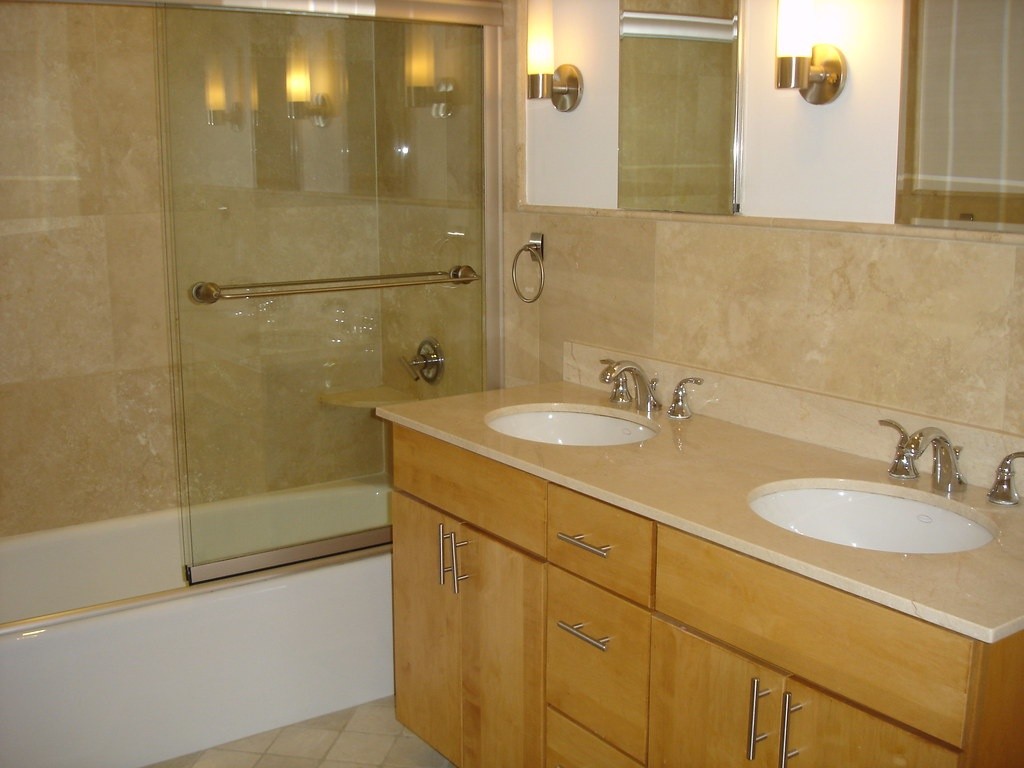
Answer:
0, 471, 459, 768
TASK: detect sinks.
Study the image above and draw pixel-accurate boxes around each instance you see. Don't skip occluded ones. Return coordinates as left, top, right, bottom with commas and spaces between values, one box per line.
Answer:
482, 403, 663, 446
744, 476, 1001, 553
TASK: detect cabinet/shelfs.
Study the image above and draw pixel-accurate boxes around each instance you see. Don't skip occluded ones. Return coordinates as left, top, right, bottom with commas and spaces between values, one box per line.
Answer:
392, 421, 1024, 768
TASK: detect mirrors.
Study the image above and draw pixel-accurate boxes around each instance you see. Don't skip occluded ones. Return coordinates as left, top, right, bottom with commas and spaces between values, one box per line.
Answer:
893, 0, 1024, 235
615, 0, 743, 213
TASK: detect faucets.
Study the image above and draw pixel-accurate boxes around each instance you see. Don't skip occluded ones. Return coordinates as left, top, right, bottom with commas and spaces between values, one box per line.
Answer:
599, 361, 662, 412
887, 427, 968, 493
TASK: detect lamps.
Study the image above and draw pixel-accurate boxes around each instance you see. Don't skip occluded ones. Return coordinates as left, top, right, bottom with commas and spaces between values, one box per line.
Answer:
528, 0, 584, 113
777, 0, 848, 105
404, 25, 458, 120
203, 52, 246, 132
284, 34, 331, 128
